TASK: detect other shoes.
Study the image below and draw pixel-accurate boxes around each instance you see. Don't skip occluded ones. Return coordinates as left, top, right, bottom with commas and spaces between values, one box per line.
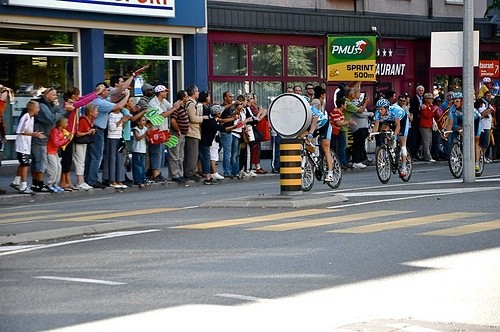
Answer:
475, 163, 480, 170
352, 162, 366, 169
483, 156, 490, 164
400, 168, 407, 177
485, 157, 493, 163
324, 174, 335, 182
0, 167, 268, 194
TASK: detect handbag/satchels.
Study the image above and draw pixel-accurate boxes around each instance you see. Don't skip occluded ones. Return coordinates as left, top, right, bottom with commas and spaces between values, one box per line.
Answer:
60, 129, 74, 151
242, 121, 256, 144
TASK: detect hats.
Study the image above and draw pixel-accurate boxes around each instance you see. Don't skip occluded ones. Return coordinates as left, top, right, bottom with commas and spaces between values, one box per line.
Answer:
483, 77, 492, 84
141, 84, 155, 92
154, 84, 170, 93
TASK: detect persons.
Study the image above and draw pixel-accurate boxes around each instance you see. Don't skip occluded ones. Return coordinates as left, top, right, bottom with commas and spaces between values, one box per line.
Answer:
301, 96, 334, 182
286, 77, 500, 169
0, 62, 279, 195
372, 99, 409, 176
443, 92, 482, 170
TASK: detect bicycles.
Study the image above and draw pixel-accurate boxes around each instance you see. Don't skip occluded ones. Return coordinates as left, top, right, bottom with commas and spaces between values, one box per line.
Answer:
369, 130, 413, 184
444, 130, 485, 178
296, 136, 343, 192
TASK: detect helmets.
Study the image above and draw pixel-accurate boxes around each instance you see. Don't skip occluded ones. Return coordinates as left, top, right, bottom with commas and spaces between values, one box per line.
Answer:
375, 99, 390, 107
452, 92, 463, 99
303, 96, 311, 103
